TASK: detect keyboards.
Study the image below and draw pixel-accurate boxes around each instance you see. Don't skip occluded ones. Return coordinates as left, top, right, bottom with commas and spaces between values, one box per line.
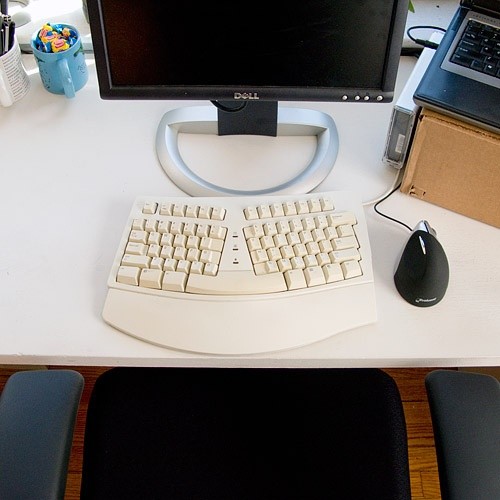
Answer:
101, 190, 378, 356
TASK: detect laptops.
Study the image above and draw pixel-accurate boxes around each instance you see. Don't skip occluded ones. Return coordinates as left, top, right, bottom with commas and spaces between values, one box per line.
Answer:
413, 0, 500, 136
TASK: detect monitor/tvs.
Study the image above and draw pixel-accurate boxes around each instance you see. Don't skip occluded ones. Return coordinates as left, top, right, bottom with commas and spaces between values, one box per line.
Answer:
86, 0, 410, 197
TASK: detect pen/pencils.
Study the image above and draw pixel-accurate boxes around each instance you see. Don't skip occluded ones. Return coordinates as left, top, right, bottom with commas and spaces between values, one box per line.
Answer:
0, 15, 15, 58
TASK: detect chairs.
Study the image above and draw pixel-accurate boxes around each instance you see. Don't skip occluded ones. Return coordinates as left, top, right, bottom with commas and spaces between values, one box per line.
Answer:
0, 368, 500, 500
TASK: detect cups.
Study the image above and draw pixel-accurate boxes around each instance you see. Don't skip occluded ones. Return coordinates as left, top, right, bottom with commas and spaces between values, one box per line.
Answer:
31, 24, 88, 98
0, 26, 30, 107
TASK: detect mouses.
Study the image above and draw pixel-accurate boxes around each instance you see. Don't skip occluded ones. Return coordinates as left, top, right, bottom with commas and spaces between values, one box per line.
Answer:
393, 219, 448, 308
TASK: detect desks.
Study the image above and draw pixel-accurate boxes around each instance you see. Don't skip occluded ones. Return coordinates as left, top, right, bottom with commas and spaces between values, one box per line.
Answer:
1, 0, 500, 367
